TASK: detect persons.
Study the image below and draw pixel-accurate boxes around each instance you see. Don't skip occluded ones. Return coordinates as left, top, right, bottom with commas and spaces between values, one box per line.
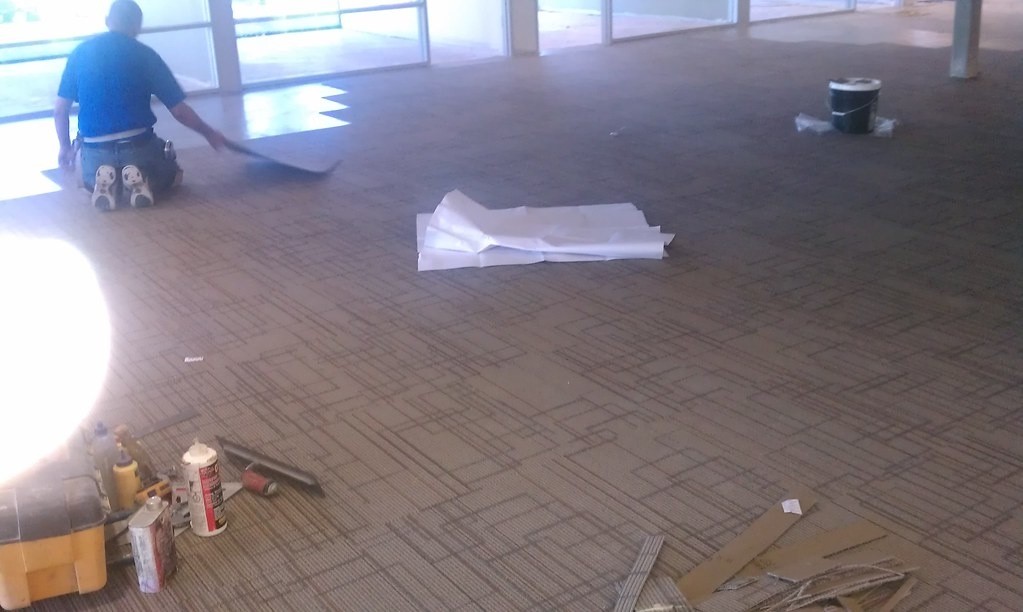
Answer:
52, 0, 226, 209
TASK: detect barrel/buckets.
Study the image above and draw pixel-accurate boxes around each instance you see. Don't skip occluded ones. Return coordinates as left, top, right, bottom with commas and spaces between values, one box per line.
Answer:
825, 77, 882, 134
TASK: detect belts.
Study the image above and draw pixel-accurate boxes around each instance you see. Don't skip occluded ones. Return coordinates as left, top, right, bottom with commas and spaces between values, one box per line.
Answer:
83, 131, 152, 151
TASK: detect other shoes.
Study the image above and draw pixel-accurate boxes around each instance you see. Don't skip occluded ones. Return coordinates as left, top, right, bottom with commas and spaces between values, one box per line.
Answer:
91, 163, 117, 212
119, 164, 154, 208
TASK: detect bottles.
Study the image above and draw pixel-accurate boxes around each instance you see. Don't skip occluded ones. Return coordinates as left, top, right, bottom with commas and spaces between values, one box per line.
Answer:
128, 496, 179, 595
180, 439, 229, 537
92, 421, 119, 511
113, 450, 140, 510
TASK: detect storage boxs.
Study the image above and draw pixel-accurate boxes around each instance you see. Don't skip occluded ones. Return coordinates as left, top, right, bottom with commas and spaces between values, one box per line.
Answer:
0, 448, 112, 612
